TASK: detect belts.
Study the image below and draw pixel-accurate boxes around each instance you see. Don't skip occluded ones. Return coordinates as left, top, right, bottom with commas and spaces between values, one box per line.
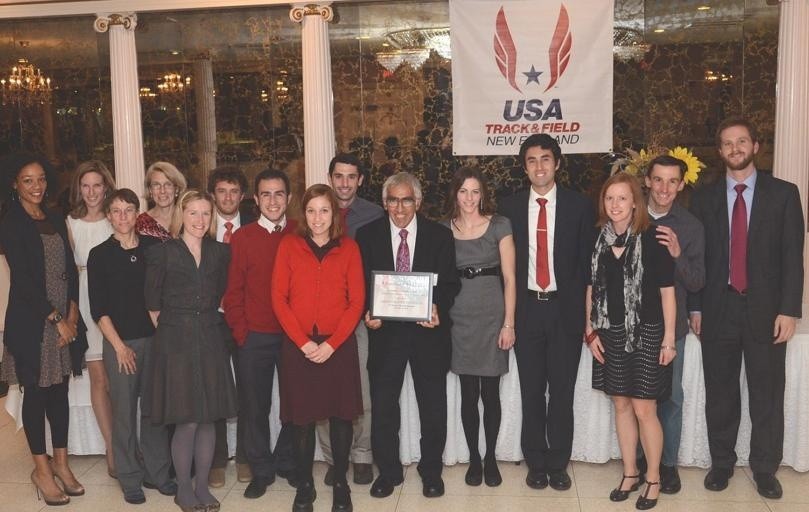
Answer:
458, 267, 500, 278
527, 290, 559, 301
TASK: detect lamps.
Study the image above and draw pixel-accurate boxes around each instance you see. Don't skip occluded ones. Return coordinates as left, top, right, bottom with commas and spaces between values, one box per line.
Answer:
613, 27, 652, 65
376, 27, 452, 73
0, 41, 54, 105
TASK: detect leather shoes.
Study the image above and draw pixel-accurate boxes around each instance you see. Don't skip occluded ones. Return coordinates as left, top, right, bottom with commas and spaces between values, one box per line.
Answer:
332, 482, 354, 512
195, 492, 220, 512
638, 478, 663, 510
754, 471, 784, 499
464, 457, 483, 487
664, 468, 681, 494
292, 482, 317, 511
610, 473, 642, 501
371, 467, 404, 497
354, 462, 373, 484
174, 495, 205, 512
279, 469, 298, 485
422, 469, 443, 495
325, 466, 335, 484
485, 458, 502, 487
245, 473, 275, 499
527, 466, 548, 489
549, 466, 572, 490
704, 466, 734, 491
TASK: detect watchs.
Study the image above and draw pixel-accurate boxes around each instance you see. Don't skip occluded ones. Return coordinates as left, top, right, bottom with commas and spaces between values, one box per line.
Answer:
50, 311, 63, 325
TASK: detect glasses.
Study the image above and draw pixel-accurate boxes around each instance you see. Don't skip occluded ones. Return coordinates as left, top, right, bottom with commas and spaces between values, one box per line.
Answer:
149, 182, 175, 189
109, 207, 136, 216
384, 197, 419, 208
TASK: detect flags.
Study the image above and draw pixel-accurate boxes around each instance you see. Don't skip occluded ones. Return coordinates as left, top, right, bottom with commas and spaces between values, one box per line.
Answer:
449, 1, 617, 156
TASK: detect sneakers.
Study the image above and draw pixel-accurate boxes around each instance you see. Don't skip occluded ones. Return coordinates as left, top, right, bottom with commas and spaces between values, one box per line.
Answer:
120, 479, 146, 504
144, 477, 176, 497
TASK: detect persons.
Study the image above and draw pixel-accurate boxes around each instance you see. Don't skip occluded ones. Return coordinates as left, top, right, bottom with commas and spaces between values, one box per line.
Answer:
354, 173, 462, 498
87, 188, 177, 504
271, 184, 365, 512
436, 166, 515, 486
316, 155, 386, 485
0, 252, 11, 398
586, 172, 677, 510
144, 191, 231, 511
208, 166, 256, 487
0, 151, 90, 505
635, 155, 706, 495
135, 162, 187, 242
688, 117, 805, 498
224, 168, 300, 499
64, 159, 119, 479
497, 135, 597, 490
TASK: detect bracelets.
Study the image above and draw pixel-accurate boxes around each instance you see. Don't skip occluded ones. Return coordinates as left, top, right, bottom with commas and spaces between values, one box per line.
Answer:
0, 330, 5, 333
502, 325, 514, 328
586, 330, 598, 348
659, 345, 676, 350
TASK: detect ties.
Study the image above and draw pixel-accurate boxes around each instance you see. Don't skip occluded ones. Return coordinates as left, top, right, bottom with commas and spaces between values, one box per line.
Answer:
224, 223, 235, 246
730, 184, 748, 294
396, 229, 411, 273
338, 208, 350, 238
536, 198, 550, 288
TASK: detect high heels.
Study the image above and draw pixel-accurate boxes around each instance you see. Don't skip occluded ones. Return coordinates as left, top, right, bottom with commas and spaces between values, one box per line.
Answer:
47, 460, 84, 496
102, 449, 118, 479
31, 469, 70, 504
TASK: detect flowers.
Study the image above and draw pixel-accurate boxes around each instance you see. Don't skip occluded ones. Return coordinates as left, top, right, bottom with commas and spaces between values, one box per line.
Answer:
601, 146, 707, 194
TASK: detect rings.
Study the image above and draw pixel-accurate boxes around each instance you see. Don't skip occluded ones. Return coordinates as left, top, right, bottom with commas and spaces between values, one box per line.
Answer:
73, 337, 76, 341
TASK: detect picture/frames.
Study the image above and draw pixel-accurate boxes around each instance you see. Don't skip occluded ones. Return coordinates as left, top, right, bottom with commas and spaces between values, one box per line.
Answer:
370, 270, 434, 322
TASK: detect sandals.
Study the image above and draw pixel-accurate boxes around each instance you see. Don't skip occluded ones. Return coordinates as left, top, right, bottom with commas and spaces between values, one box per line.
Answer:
209, 467, 226, 487
236, 462, 252, 480
